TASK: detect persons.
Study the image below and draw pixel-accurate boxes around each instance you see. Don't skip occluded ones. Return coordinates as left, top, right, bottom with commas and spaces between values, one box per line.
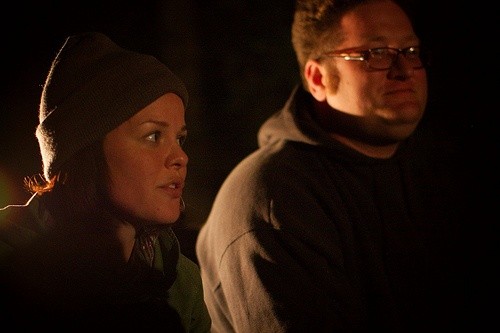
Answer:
195, 0, 460, 333
0, 33, 210, 333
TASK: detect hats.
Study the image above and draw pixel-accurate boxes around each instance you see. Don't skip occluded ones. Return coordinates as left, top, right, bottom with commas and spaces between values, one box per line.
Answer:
34, 32, 189, 180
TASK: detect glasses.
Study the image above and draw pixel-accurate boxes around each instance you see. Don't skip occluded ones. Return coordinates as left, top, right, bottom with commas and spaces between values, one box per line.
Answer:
321, 46, 428, 69
